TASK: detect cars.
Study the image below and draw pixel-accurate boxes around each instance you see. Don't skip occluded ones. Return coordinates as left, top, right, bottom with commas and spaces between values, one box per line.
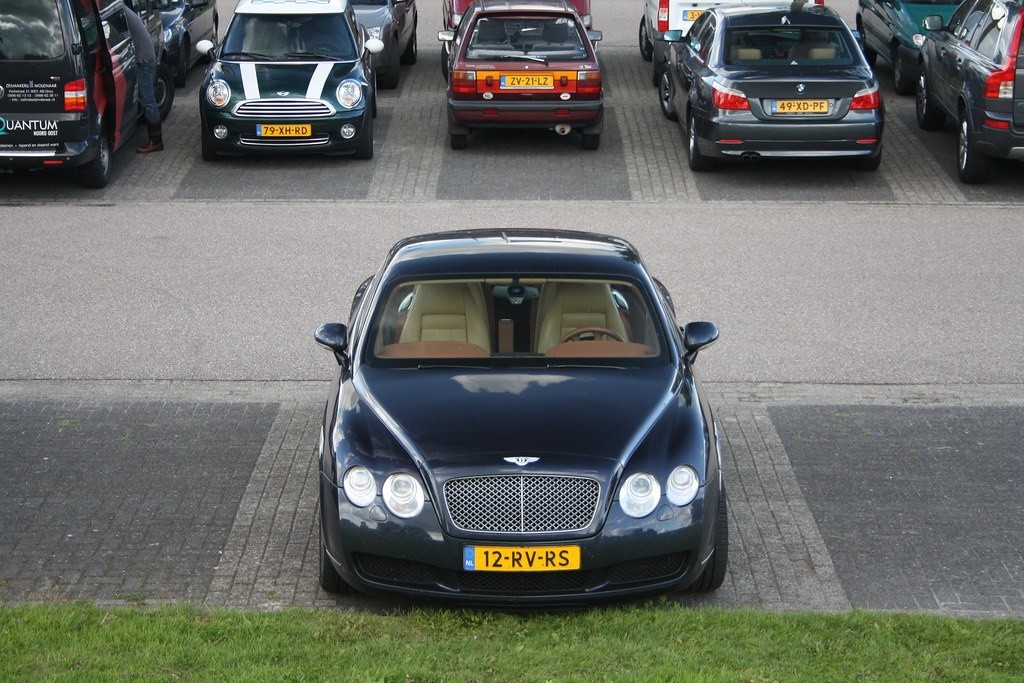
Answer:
351, 0, 419, 92
308, 224, 732, 615
658, 2, 887, 173
442, 0, 594, 87
639, 0, 826, 89
438, 1, 605, 153
154, 0, 219, 89
194, 0, 385, 162
854, 0, 964, 94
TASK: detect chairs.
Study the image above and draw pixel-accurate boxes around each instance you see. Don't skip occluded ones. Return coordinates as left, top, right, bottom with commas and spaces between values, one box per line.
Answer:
731, 45, 762, 59
534, 281, 631, 354
471, 19, 514, 50
788, 40, 840, 60
241, 17, 290, 57
398, 282, 492, 355
532, 22, 576, 50
299, 19, 349, 55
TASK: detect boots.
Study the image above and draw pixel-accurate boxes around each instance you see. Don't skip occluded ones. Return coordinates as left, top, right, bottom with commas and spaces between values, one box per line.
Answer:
137, 120, 164, 153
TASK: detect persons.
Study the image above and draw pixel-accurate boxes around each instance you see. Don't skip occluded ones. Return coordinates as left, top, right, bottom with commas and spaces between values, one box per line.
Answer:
124, 4, 165, 154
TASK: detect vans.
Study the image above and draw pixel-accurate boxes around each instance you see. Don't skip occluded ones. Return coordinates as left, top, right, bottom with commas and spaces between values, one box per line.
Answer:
0, 0, 177, 192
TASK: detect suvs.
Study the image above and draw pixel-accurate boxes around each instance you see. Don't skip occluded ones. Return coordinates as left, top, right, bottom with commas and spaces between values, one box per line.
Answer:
914, 0, 1024, 186
